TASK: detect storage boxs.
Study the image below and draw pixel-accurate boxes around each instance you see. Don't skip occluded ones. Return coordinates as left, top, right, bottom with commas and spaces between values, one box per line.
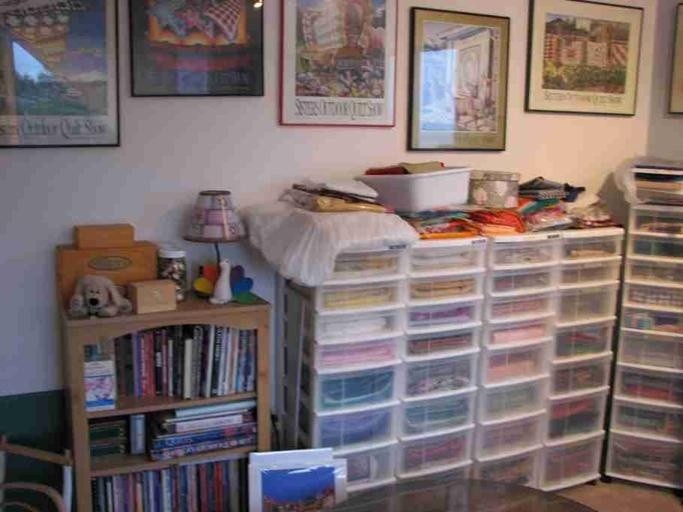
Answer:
276, 162, 683, 497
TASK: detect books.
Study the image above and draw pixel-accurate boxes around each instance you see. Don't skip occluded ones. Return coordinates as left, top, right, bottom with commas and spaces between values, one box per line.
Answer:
85, 325, 259, 512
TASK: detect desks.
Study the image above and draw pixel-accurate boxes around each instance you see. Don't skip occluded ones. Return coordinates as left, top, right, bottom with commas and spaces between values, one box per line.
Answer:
326, 471, 600, 511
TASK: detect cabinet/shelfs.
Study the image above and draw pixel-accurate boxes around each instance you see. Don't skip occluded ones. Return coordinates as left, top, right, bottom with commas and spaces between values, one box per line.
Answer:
57, 289, 275, 512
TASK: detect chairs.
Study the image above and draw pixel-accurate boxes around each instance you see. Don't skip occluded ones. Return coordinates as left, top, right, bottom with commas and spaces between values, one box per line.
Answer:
1, 430, 77, 512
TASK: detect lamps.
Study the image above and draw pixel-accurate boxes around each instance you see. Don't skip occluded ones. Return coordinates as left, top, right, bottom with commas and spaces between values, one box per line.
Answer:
179, 187, 250, 279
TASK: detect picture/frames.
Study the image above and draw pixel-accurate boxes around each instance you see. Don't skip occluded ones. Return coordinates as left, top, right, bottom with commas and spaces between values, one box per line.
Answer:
277, 1, 398, 128
525, 1, 644, 117
128, 2, 264, 97
669, 1, 683, 114
406, 6, 511, 151
1, 2, 121, 149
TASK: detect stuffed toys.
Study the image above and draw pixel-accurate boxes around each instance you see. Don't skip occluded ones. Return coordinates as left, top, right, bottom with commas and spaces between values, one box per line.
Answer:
191, 259, 260, 306
65, 269, 132, 317
